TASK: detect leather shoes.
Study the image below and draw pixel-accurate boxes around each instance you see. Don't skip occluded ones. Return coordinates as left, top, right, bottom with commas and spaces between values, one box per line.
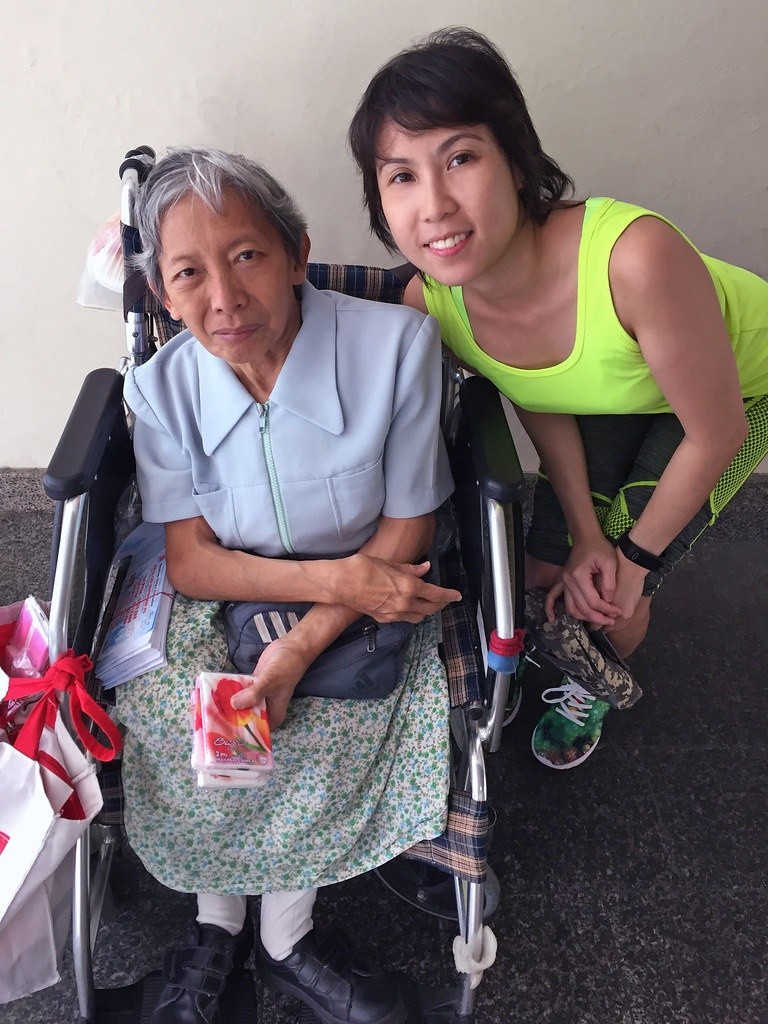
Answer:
151, 899, 254, 1024
255, 916, 418, 1024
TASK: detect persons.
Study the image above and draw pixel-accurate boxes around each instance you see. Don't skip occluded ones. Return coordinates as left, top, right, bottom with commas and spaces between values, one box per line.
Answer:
114, 149, 461, 1024
349, 27, 768, 771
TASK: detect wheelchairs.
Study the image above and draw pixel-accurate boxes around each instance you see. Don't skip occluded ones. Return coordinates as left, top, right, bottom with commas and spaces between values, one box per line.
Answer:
38, 145, 524, 1024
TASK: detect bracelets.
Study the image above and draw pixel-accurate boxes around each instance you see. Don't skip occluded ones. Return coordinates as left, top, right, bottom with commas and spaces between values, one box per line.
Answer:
616, 531, 663, 572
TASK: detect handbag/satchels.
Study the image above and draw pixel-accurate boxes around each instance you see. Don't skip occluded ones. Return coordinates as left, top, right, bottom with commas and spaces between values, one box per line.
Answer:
1, 594, 123, 1009
221, 602, 417, 701
521, 584, 644, 711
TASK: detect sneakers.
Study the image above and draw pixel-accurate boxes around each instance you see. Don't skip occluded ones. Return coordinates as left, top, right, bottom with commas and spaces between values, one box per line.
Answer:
531, 671, 610, 770
484, 641, 541, 727
